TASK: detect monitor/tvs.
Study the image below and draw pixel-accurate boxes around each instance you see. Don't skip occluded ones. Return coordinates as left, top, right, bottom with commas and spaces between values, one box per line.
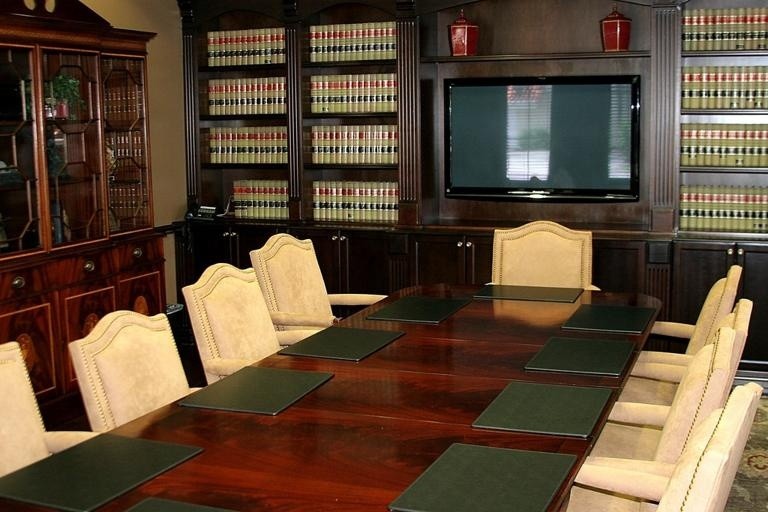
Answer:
443, 75, 642, 204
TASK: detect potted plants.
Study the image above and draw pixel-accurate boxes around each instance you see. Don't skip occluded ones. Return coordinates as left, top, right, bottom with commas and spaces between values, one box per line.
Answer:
42, 74, 85, 120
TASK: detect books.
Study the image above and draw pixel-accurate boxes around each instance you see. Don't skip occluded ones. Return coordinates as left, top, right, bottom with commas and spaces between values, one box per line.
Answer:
104, 81, 145, 223
205, 126, 288, 164
209, 77, 288, 115
309, 22, 397, 62
208, 28, 285, 67
311, 73, 398, 113
233, 179, 289, 219
312, 125, 398, 164
678, 5, 767, 234
313, 181, 399, 220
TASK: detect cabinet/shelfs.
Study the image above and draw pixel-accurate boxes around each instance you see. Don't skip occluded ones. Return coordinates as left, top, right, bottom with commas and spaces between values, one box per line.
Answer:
180, 3, 421, 229
680, 6, 768, 241
2, 2, 173, 404
